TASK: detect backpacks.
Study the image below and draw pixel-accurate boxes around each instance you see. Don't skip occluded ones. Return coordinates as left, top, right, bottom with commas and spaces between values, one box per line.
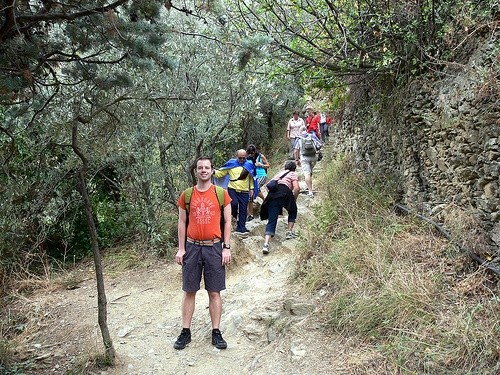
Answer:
295, 136, 316, 156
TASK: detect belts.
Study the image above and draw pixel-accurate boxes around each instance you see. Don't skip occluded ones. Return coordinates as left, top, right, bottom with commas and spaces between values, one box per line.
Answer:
186, 237, 221, 246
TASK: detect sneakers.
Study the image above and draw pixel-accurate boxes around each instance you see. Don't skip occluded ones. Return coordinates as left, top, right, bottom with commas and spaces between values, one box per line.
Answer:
174, 331, 191, 349
286, 232, 298, 238
262, 243, 269, 253
212, 329, 227, 347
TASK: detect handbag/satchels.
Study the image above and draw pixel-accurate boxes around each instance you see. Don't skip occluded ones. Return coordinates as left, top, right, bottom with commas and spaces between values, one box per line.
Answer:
266, 180, 278, 191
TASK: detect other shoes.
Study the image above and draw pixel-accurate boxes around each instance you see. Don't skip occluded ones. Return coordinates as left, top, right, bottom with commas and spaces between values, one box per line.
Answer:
248, 215, 254, 221
309, 192, 314, 198
236, 227, 250, 233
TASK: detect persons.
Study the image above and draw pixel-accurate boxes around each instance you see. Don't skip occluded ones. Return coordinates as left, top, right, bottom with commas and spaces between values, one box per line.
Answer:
287, 107, 334, 198
259, 161, 301, 254
174, 156, 232, 349
209, 144, 271, 232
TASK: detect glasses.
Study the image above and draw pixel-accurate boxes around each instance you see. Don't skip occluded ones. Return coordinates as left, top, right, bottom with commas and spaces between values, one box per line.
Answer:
237, 156, 245, 160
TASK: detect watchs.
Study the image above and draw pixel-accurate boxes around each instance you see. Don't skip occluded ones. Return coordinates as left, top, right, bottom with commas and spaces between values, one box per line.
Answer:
222, 244, 230, 249
